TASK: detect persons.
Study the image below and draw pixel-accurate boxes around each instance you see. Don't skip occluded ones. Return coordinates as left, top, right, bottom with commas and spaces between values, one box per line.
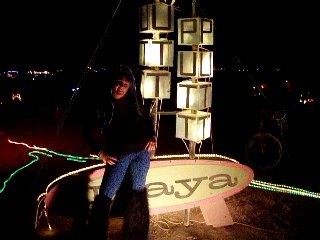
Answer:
85, 63, 157, 240
35, 174, 91, 240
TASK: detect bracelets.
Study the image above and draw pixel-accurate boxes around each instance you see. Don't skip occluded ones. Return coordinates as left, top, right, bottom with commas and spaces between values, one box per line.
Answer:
97, 149, 104, 156
